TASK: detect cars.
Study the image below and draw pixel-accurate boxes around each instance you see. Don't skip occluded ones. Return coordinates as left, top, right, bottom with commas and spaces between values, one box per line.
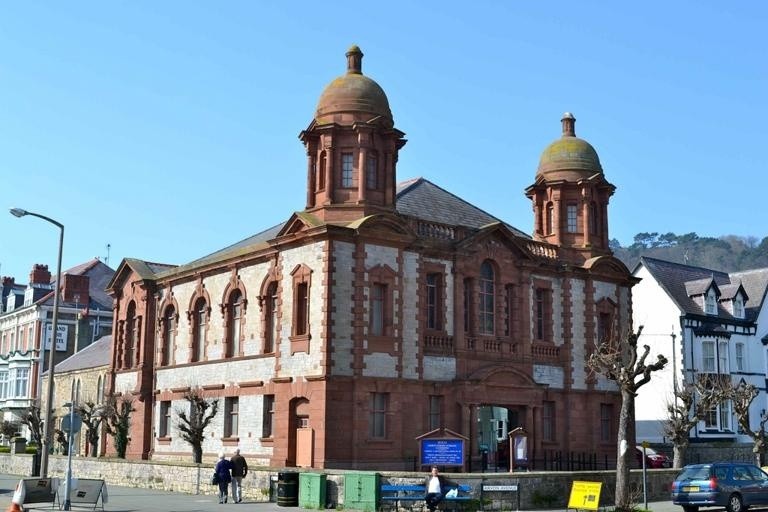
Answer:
670, 460, 768, 511
636, 446, 671, 469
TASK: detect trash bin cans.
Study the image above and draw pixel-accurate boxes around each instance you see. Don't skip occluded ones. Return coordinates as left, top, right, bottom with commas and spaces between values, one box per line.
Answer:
9, 437, 27, 454
277, 472, 299, 507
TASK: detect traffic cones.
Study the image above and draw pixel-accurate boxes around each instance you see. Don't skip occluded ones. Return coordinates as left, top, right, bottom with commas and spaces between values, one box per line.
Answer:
6, 483, 21, 512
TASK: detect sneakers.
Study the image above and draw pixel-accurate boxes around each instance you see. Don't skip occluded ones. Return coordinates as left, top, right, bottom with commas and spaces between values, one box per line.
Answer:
219, 497, 242, 504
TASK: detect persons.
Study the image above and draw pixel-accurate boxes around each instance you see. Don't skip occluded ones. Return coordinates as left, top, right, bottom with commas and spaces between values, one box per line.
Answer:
408, 465, 464, 511
228, 448, 248, 503
214, 451, 231, 503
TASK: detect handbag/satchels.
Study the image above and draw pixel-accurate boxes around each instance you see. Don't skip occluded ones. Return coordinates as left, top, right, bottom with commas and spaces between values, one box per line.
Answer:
212, 474, 217, 485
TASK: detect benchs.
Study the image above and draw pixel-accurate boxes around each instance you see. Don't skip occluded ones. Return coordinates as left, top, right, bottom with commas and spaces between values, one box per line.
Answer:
381, 485, 471, 512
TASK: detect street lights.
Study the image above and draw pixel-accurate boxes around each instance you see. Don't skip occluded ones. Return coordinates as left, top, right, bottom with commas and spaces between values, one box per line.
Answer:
8, 208, 65, 481
